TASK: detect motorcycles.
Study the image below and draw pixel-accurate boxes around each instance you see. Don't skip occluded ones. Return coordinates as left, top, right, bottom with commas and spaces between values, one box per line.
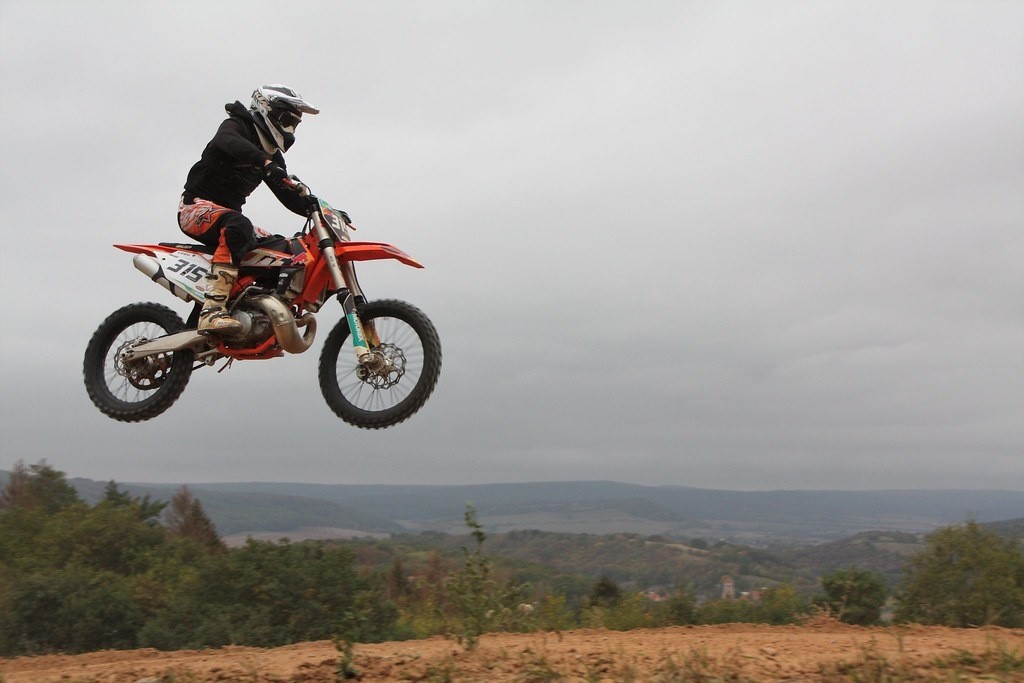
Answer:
82, 175, 442, 429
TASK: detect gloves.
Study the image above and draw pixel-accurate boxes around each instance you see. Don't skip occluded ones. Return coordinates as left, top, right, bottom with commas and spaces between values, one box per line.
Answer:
335, 209, 351, 225
263, 164, 289, 187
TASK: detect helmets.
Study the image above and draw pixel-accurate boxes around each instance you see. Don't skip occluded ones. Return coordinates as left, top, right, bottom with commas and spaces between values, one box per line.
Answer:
250, 86, 319, 154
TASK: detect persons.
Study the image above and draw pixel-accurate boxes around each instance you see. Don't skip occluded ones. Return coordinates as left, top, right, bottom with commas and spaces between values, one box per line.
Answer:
177, 83, 352, 336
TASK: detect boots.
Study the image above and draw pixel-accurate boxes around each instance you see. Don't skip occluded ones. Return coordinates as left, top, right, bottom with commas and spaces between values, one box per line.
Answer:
198, 264, 242, 335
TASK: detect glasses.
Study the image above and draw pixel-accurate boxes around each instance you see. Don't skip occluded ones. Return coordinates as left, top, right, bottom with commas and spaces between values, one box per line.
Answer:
278, 111, 302, 134
